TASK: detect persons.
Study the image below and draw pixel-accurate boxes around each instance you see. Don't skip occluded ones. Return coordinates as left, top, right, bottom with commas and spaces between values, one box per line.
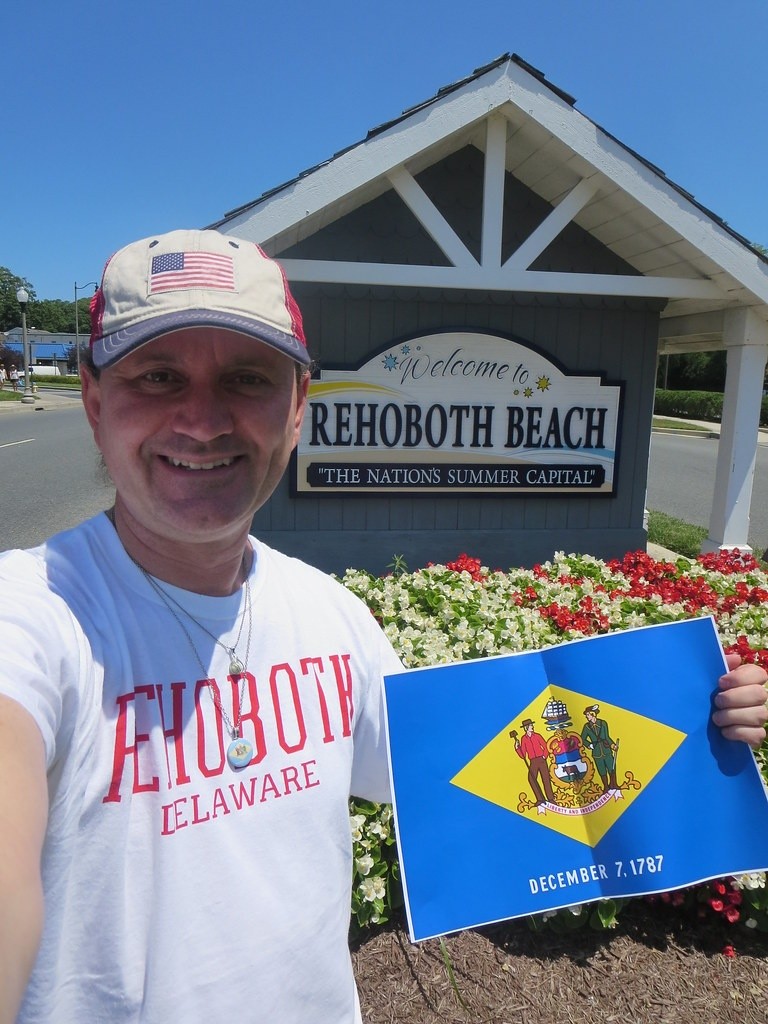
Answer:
0, 363, 20, 392
0, 229, 768, 1024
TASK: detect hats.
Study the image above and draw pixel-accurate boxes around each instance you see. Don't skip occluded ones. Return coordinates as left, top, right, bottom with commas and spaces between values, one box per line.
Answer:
10, 365, 18, 369
0, 364, 5, 367
89, 228, 311, 373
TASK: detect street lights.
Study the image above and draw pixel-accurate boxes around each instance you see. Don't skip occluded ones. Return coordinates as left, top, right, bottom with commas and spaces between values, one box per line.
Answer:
74, 281, 100, 377
16, 287, 36, 405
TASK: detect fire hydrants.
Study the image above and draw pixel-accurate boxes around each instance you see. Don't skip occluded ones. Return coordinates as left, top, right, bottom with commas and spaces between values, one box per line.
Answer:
33, 383, 38, 393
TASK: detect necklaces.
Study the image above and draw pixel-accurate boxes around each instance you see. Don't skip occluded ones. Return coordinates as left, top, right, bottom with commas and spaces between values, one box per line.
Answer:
108, 504, 252, 767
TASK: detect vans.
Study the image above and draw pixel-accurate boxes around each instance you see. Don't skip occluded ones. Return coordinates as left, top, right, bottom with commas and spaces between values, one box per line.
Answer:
16, 365, 61, 382
